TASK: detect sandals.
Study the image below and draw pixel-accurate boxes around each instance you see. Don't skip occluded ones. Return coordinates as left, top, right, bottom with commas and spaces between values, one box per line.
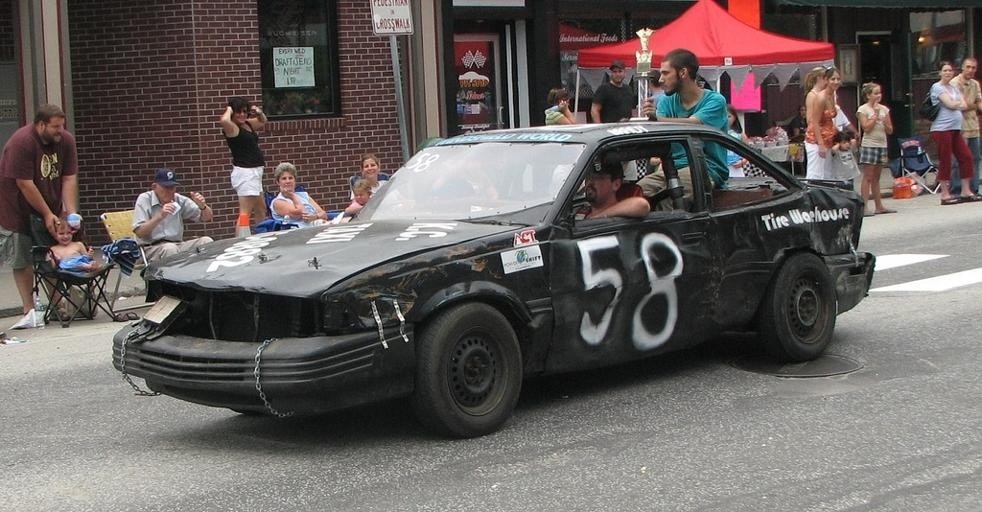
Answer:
942, 194, 982, 205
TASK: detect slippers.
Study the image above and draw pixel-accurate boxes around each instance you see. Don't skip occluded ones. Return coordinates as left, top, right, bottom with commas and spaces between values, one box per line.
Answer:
114, 312, 140, 322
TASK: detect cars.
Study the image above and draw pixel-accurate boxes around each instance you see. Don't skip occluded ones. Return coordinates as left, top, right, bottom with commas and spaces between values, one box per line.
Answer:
120, 118, 883, 432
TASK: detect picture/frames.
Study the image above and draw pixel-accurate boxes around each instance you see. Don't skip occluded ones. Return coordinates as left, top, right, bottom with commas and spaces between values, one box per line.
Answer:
836, 43, 862, 88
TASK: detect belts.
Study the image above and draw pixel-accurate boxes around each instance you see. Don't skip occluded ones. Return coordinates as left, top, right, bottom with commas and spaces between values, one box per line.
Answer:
144, 239, 181, 248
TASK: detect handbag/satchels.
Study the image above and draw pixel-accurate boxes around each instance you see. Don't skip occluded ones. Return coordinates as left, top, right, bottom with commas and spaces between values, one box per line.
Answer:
920, 97, 942, 121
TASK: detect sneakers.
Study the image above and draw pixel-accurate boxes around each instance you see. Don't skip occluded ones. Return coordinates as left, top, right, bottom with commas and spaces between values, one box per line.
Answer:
50, 306, 69, 321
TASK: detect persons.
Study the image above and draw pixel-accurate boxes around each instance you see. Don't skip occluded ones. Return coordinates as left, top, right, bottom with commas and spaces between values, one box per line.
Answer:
544, 87, 577, 126
569, 160, 650, 219
763, 65, 859, 187
948, 55, 981, 201
648, 69, 666, 106
725, 102, 749, 178
133, 166, 216, 266
0, 103, 78, 322
854, 82, 898, 217
928, 60, 982, 205
635, 48, 729, 211
591, 55, 656, 123
50, 216, 100, 272
269, 153, 390, 230
220, 96, 268, 237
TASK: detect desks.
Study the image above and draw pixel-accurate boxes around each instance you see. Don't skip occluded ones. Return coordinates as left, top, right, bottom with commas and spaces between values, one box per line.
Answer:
755, 143, 806, 177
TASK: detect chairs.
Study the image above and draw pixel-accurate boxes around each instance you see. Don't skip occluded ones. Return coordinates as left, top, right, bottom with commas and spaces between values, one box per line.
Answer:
350, 173, 389, 189
615, 183, 644, 201
710, 186, 772, 206
900, 136, 941, 195
100, 209, 157, 313
254, 184, 340, 233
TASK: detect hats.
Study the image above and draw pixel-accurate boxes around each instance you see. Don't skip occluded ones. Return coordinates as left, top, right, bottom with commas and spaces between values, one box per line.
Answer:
590, 161, 623, 178
610, 60, 626, 69
153, 166, 180, 186
